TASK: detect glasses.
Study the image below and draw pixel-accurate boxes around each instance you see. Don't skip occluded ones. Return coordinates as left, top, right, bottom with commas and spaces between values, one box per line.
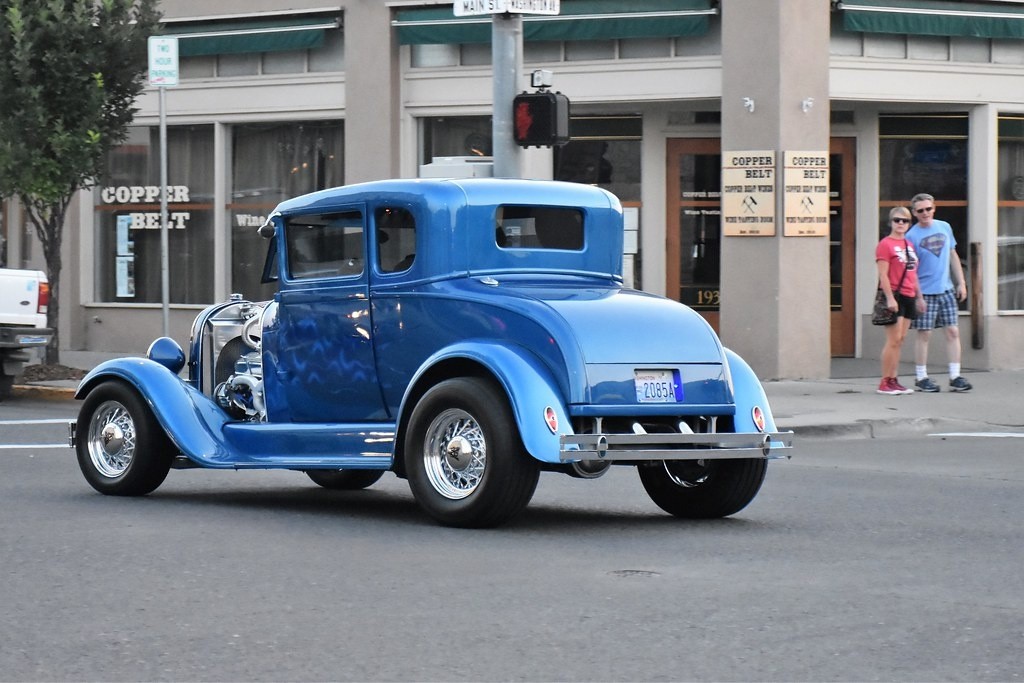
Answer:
914, 206, 936, 213
890, 217, 911, 223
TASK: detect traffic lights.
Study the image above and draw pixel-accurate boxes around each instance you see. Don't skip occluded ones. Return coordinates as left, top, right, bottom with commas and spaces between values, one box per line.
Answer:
513, 92, 572, 147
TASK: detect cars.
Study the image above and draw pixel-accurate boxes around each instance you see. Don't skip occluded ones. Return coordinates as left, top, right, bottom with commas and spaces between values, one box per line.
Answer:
67, 177, 796, 530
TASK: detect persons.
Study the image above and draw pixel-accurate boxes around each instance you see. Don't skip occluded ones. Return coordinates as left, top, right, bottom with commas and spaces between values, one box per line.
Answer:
874, 206, 927, 396
903, 193, 974, 392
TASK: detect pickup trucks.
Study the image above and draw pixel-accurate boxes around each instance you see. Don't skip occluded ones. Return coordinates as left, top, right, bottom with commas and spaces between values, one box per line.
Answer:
0, 268, 55, 401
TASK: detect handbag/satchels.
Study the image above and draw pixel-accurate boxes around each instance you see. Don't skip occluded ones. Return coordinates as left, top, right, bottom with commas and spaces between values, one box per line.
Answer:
871, 289, 899, 325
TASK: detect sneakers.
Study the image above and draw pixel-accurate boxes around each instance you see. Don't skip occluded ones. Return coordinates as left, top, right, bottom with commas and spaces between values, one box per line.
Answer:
914, 378, 941, 392
876, 376, 901, 394
889, 377, 914, 393
949, 376, 972, 391
